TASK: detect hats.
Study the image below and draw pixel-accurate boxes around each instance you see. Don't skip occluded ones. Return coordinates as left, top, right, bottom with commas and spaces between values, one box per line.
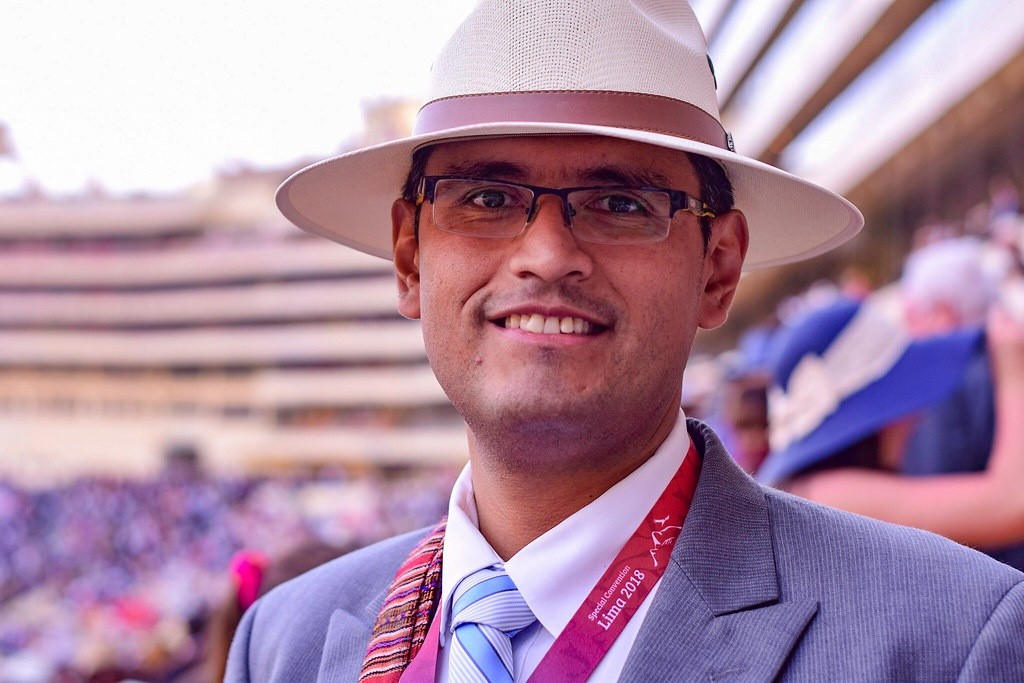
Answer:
275, 0, 864, 272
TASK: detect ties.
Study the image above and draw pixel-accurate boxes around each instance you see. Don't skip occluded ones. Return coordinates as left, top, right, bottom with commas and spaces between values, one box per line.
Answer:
442, 564, 534, 683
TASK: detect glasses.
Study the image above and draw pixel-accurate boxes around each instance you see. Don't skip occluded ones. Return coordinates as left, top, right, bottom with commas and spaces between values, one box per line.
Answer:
411, 174, 714, 246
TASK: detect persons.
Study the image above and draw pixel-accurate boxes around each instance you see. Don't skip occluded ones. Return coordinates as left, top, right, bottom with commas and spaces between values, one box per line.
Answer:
221, 3, 1023, 683
680, 171, 1024, 550
0, 463, 458, 683
